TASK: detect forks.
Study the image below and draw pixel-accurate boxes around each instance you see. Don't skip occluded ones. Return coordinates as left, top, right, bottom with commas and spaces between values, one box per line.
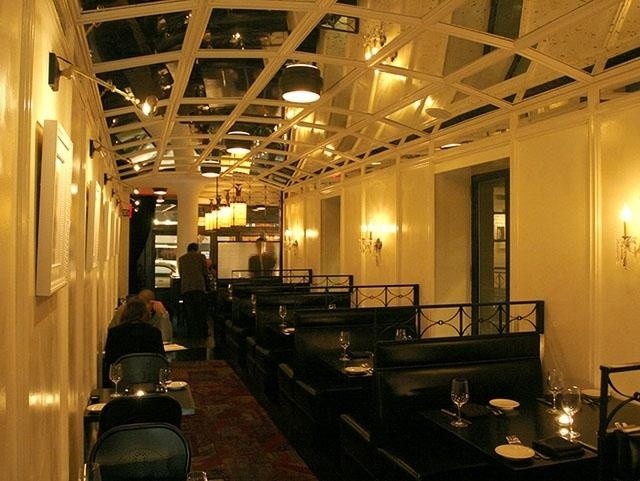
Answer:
504, 434, 551, 461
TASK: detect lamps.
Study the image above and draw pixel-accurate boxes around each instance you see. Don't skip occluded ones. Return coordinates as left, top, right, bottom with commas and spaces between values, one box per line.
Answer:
230, 200, 247, 227
153, 187, 167, 195
617, 204, 640, 270
49, 52, 159, 117
205, 212, 213, 230
112, 189, 140, 206
90, 139, 143, 174
359, 224, 383, 266
104, 173, 140, 195
284, 234, 298, 255
201, 161, 221, 177
217, 204, 230, 229
366, 25, 396, 73
226, 132, 250, 153
116, 200, 139, 213
282, 64, 320, 103
212, 209, 219, 229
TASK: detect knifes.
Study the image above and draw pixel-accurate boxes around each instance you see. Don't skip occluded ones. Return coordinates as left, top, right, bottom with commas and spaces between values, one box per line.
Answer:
441, 408, 473, 426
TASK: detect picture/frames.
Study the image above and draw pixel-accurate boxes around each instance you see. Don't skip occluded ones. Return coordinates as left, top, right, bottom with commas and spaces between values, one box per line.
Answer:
86, 181, 101, 269
104, 201, 110, 262
116, 218, 120, 254
36, 120, 73, 296
111, 212, 116, 257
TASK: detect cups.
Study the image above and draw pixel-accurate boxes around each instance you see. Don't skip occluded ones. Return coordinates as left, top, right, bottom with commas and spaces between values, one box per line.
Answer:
278, 306, 287, 328
228, 284, 233, 300
560, 387, 581, 440
158, 368, 173, 395
251, 294, 257, 312
448, 379, 470, 427
545, 367, 565, 416
394, 329, 406, 343
109, 364, 123, 398
328, 304, 337, 309
338, 331, 352, 362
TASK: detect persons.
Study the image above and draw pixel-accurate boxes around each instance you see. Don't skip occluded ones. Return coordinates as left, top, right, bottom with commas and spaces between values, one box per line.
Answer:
102, 295, 169, 380
178, 243, 214, 347
109, 290, 174, 347
248, 238, 276, 277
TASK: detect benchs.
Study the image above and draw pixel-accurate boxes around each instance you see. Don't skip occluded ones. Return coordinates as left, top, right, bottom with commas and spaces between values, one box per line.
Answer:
256, 275, 353, 336
599, 365, 640, 481
217, 277, 282, 296
294, 284, 420, 374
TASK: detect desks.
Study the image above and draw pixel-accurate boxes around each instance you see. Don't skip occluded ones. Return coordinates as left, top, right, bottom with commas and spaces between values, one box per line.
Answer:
232, 270, 311, 300
313, 353, 376, 397
418, 394, 640, 481
170, 275, 181, 312
85, 383, 195, 464
102, 341, 189, 387
264, 320, 295, 349
375, 302, 544, 422
236, 299, 255, 335
106, 352, 171, 387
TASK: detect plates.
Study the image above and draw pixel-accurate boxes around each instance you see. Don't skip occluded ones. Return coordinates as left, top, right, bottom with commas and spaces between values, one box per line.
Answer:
489, 399, 519, 410
579, 389, 612, 402
86, 403, 108, 413
494, 443, 537, 459
344, 366, 370, 374
166, 382, 188, 392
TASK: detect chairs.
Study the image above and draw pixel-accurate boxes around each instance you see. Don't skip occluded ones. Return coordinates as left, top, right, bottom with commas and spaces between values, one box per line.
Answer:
87, 423, 192, 481
98, 395, 182, 436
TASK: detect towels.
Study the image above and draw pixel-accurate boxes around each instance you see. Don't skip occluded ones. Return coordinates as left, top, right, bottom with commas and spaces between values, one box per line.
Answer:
350, 350, 370, 359
532, 431, 586, 461
